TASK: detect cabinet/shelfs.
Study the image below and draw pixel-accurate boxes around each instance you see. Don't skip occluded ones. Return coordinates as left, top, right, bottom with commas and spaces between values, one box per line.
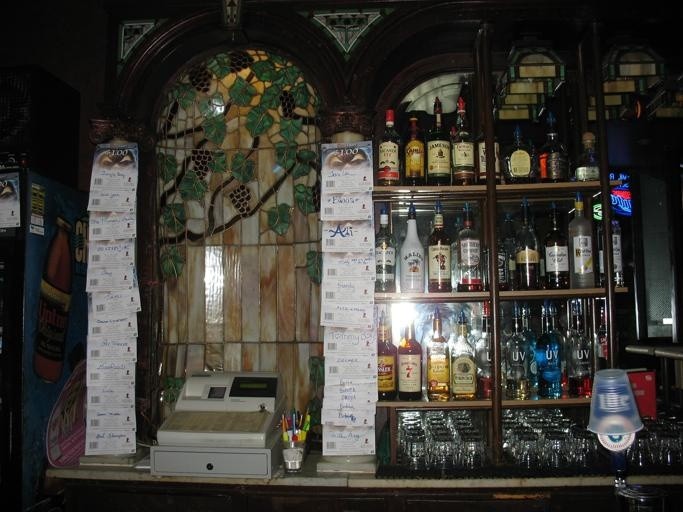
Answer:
319, 11, 627, 475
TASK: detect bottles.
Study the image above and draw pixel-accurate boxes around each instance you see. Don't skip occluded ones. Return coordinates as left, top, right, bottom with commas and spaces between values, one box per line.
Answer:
405, 112, 423, 186
425, 97, 452, 184
502, 212, 521, 289
399, 204, 426, 293
567, 297, 590, 396
500, 125, 538, 184
375, 206, 395, 294
505, 305, 530, 399
451, 311, 479, 401
486, 240, 509, 291
425, 306, 451, 401
574, 132, 600, 181
538, 109, 571, 182
522, 305, 537, 391
535, 300, 562, 399
397, 322, 421, 402
457, 203, 483, 291
543, 201, 571, 288
427, 200, 451, 293
33, 217, 73, 382
595, 305, 608, 369
377, 312, 394, 400
451, 98, 476, 185
516, 196, 541, 288
567, 192, 596, 288
375, 109, 404, 185
476, 302, 502, 400
598, 218, 624, 288
475, 120, 502, 184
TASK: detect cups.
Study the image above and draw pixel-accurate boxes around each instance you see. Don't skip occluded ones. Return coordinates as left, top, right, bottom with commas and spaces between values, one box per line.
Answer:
647, 412, 682, 435
628, 435, 682, 466
501, 409, 596, 471
614, 485, 666, 511
280, 441, 306, 473
586, 369, 645, 435
398, 412, 485, 473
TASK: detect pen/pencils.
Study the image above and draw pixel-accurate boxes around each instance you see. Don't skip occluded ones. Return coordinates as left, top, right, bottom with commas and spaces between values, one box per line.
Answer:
280, 407, 312, 441
